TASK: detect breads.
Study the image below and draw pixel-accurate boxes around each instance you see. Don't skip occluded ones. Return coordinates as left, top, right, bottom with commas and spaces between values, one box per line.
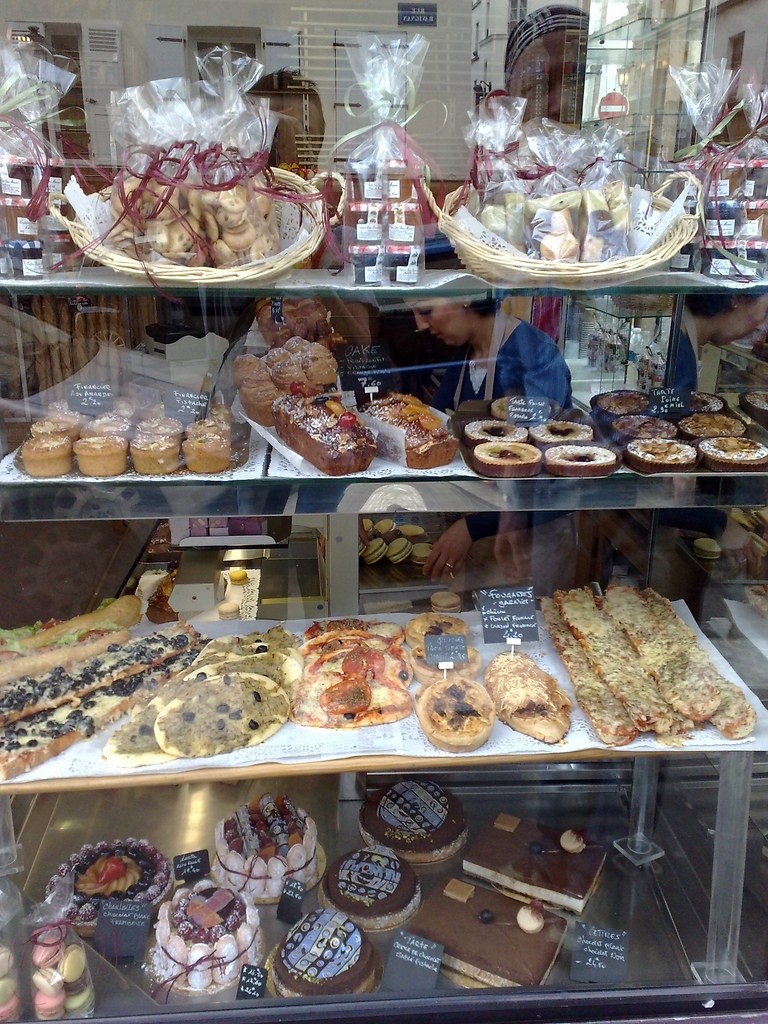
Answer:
21, 299, 768, 476
0, 518, 768, 780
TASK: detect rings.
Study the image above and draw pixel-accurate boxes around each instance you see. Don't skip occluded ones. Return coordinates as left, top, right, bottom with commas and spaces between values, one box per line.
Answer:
739, 557, 747, 564
446, 562, 454, 569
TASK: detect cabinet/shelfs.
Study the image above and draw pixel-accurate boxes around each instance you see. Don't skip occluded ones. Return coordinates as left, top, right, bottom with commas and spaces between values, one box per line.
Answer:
0, 266, 768, 1023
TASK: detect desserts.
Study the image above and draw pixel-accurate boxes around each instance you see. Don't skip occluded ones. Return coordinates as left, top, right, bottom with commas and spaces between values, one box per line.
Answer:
479, 182, 631, 263
0, 932, 95, 1023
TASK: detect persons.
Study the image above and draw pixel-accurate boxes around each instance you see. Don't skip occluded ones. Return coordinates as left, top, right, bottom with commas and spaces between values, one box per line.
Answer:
403, 296, 573, 586
658, 292, 768, 581
180, 63, 374, 549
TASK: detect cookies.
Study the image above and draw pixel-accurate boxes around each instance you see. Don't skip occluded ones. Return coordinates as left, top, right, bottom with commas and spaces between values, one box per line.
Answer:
111, 177, 279, 268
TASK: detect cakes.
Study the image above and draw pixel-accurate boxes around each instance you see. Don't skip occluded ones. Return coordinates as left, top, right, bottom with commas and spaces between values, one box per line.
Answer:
148, 880, 264, 992
359, 778, 468, 861
49, 839, 176, 939
405, 876, 567, 987
211, 792, 316, 899
464, 812, 607, 914
320, 850, 422, 932
270, 907, 375, 999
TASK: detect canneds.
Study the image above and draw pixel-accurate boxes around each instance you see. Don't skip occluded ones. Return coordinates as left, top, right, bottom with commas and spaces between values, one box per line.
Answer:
345, 161, 421, 285
0, 155, 72, 279
666, 158, 768, 281
